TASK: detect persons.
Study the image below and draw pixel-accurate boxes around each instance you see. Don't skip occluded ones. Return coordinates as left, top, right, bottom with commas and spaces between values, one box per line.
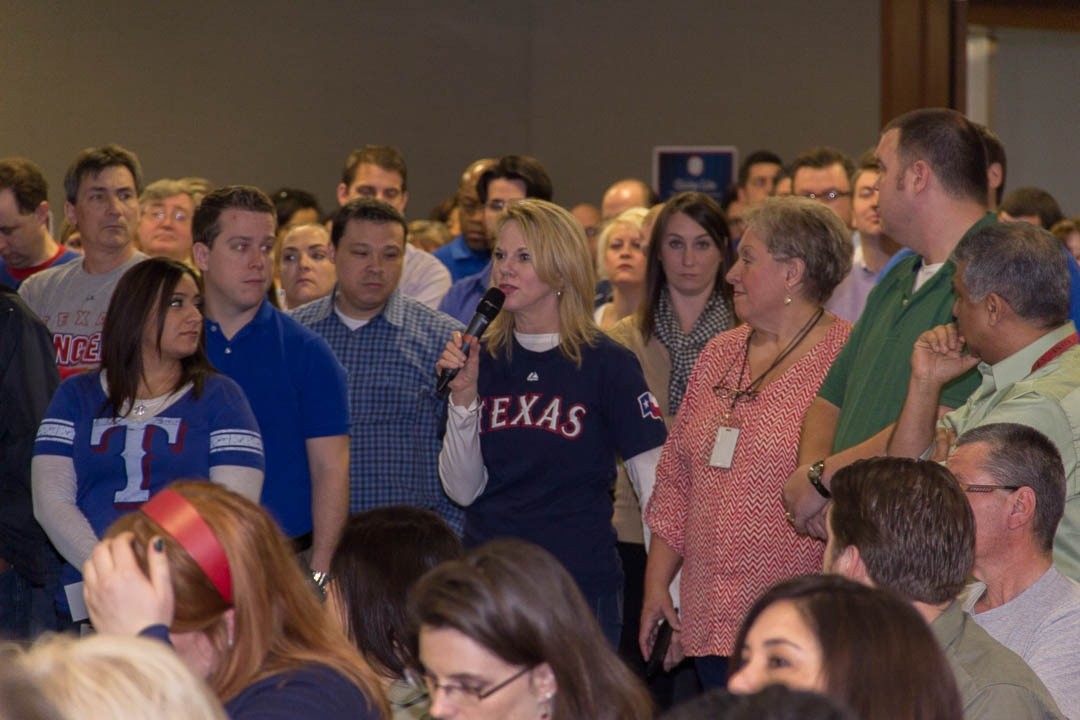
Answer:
0, 108, 1080, 720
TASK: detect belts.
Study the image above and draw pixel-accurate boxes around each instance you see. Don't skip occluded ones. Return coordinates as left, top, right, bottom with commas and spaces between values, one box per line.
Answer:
284, 534, 315, 554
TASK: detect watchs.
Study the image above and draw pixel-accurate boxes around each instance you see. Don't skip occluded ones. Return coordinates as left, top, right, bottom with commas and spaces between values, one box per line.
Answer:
807, 459, 832, 499
310, 569, 331, 599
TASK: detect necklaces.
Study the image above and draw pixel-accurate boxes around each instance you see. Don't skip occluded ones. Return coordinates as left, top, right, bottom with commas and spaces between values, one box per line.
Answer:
133, 370, 174, 415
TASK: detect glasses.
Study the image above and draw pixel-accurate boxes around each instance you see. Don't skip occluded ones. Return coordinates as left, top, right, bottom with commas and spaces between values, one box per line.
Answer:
141, 208, 193, 226
804, 190, 850, 202
405, 667, 533, 707
485, 199, 515, 213
272, 189, 299, 204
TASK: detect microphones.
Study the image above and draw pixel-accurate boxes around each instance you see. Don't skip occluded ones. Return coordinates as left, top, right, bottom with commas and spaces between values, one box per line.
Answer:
435, 286, 506, 395
645, 607, 679, 679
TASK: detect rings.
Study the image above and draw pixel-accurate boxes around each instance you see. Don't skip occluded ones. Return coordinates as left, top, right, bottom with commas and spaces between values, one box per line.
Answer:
786, 512, 794, 523
